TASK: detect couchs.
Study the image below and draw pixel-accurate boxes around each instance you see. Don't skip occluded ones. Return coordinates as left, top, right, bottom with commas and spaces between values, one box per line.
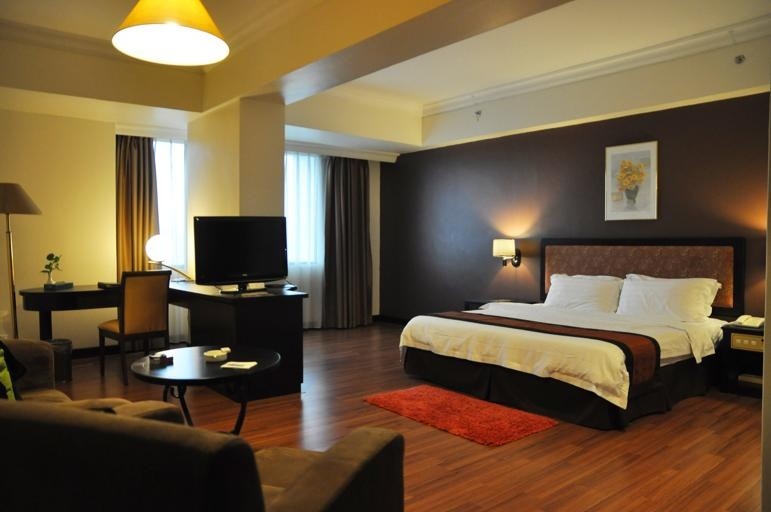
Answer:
0, 336, 405, 511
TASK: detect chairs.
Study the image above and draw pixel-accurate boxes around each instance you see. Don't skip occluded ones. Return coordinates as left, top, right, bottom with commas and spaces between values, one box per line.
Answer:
97, 270, 173, 385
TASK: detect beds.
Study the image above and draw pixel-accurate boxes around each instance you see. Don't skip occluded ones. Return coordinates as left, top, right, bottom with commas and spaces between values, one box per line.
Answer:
404, 236, 748, 430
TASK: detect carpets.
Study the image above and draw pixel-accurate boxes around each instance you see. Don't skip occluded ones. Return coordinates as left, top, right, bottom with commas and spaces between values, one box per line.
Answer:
363, 382, 561, 447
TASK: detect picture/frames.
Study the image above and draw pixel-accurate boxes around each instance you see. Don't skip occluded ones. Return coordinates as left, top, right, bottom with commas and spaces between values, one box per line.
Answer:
606, 141, 657, 222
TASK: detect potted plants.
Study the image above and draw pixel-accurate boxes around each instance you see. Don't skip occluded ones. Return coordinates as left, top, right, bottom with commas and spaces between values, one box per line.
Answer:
41, 252, 64, 285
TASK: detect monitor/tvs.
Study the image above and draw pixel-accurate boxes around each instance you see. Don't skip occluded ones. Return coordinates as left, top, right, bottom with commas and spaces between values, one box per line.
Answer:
194, 215, 289, 296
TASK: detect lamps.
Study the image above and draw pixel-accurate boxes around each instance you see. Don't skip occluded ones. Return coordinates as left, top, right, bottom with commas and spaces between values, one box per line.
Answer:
146, 234, 192, 280
492, 238, 521, 268
111, 0, 230, 68
1, 183, 43, 338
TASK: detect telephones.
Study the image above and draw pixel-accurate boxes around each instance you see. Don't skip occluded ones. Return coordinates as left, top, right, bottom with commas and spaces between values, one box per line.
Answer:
735, 313, 765, 328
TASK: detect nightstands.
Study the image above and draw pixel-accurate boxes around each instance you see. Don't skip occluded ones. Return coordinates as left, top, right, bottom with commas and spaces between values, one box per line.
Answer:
721, 318, 764, 401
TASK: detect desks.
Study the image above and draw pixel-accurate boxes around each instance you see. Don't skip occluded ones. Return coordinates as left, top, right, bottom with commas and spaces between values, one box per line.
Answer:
19, 276, 309, 402
134, 345, 283, 435
